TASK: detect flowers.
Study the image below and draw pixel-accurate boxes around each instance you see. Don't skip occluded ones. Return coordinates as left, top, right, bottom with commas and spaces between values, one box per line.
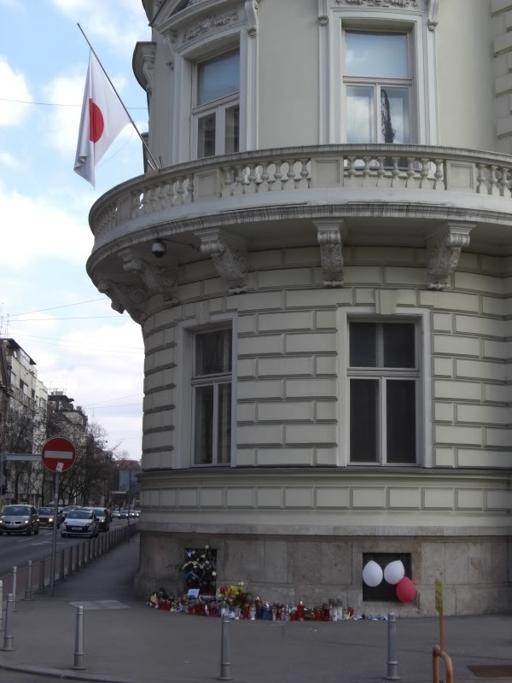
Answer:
166, 543, 217, 587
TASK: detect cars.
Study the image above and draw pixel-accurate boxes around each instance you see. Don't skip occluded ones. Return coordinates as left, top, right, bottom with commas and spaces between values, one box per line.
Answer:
111, 508, 141, 520
36, 505, 56, 530
56, 504, 112, 538
0, 503, 40, 536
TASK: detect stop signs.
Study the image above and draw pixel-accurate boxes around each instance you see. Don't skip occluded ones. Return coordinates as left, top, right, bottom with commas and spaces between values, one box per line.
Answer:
41, 435, 77, 472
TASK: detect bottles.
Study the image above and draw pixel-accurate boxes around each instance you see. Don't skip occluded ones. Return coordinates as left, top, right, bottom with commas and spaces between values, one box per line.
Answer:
219, 596, 355, 624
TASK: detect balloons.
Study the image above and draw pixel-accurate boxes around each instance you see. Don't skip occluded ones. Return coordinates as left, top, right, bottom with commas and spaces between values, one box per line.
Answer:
362, 560, 383, 589
383, 559, 406, 585
395, 576, 417, 603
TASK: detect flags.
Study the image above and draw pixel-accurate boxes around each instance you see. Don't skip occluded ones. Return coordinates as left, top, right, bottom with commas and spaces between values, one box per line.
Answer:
73, 50, 130, 189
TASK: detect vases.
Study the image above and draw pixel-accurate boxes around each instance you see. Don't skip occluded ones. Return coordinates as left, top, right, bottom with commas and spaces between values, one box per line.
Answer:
200, 584, 214, 595
